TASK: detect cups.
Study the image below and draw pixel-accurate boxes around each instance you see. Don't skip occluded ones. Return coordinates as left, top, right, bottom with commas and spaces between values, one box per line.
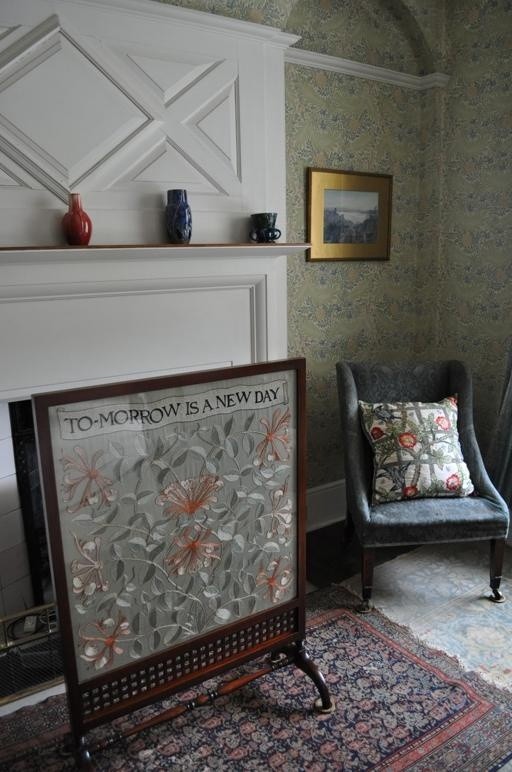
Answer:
249, 213, 281, 243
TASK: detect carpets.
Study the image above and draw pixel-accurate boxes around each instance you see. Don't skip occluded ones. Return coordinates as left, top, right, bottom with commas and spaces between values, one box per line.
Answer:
0, 577, 512, 772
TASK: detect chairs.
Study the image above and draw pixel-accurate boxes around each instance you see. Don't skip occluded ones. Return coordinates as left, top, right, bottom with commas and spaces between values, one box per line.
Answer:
335, 360, 510, 614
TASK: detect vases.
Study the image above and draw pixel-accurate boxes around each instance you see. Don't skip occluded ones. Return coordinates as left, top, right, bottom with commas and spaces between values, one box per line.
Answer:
63, 194, 92, 243
164, 189, 191, 245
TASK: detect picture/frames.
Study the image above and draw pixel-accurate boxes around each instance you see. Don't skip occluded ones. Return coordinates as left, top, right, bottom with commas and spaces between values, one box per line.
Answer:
307, 168, 393, 262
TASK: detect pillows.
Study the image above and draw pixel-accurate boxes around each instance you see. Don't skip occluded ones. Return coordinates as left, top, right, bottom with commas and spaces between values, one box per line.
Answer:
360, 391, 475, 508
